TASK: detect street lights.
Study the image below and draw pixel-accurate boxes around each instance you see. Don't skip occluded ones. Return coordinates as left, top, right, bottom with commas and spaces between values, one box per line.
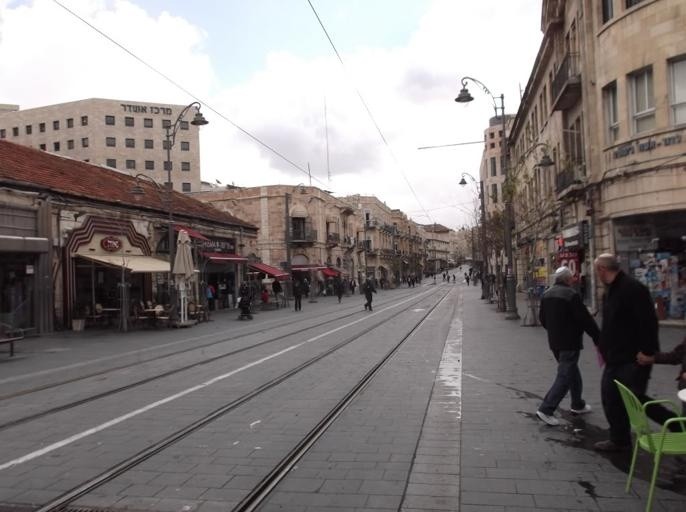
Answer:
462, 224, 479, 287
458, 171, 489, 302
128, 101, 208, 327
454, 75, 557, 321
285, 182, 309, 299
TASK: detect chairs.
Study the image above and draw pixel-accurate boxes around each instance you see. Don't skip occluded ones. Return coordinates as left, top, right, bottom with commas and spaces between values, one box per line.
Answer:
613, 379, 686, 512
133, 299, 209, 329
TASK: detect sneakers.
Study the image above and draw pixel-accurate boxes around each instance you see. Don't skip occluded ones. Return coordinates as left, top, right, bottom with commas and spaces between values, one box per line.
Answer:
535, 408, 560, 427
568, 402, 594, 415
594, 437, 632, 454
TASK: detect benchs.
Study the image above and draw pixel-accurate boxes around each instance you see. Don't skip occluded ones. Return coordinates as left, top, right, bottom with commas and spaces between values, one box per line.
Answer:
0, 323, 24, 355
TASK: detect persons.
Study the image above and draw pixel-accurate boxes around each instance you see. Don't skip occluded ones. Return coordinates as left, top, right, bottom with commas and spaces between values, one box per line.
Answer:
205, 282, 216, 311
427, 269, 472, 286
640, 338, 686, 428
238, 293, 254, 319
536, 266, 602, 426
593, 253, 659, 452
261, 274, 419, 312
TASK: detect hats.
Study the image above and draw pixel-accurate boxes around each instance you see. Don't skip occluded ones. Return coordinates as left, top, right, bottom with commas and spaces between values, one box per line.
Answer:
553, 265, 573, 284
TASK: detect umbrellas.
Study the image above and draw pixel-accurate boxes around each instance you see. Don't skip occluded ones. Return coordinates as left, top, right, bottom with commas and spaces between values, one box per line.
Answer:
171, 229, 194, 322
